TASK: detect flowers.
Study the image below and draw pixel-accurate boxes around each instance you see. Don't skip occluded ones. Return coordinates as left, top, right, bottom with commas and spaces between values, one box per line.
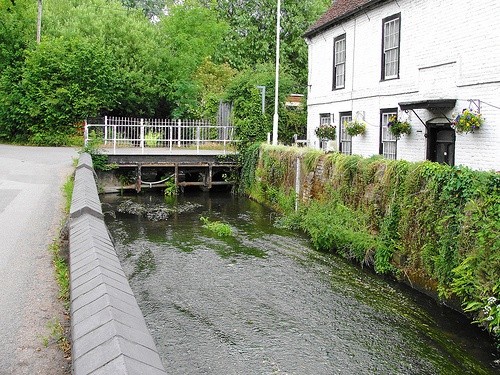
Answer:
387, 115, 412, 140
344, 120, 366, 137
315, 124, 337, 140
450, 108, 486, 134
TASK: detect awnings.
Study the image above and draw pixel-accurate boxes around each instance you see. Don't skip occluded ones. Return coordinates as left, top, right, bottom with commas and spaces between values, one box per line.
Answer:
398, 99, 456, 139
